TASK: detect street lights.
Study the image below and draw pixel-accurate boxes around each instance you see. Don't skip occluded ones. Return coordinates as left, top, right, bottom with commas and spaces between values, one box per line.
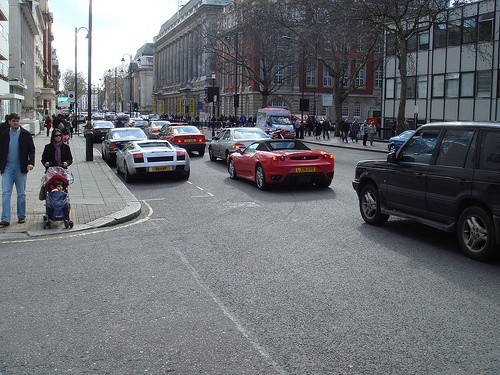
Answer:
74, 26, 92, 133
212, 71, 216, 136
105, 53, 142, 117
282, 35, 306, 119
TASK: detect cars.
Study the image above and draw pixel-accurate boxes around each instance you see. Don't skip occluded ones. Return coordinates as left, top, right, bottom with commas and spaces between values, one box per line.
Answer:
292, 114, 332, 132
76, 109, 188, 144
101, 127, 148, 165
387, 129, 437, 156
156, 122, 189, 139
141, 121, 172, 140
158, 125, 207, 156
209, 127, 273, 165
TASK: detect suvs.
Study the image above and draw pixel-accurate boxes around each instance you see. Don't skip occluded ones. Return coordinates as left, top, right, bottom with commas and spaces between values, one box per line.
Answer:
352, 121, 500, 262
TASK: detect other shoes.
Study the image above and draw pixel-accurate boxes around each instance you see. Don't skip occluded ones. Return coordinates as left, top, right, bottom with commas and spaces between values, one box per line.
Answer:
17, 217, 25, 224
0, 220, 10, 228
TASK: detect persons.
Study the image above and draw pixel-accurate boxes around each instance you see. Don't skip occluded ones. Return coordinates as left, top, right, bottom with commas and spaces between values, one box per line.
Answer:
51, 181, 63, 192
0, 115, 11, 129
0, 112, 35, 227
292, 111, 380, 147
44, 113, 77, 144
161, 112, 258, 129
41, 129, 73, 172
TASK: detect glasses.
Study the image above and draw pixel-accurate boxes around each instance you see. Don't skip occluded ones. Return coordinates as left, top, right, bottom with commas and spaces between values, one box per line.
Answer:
54, 134, 62, 138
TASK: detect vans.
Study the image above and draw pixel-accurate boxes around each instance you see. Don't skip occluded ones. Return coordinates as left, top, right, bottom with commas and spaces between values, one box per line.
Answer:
255, 108, 296, 140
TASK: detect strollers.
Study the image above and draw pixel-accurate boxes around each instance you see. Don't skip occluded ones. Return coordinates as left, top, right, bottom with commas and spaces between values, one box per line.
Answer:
43, 161, 74, 229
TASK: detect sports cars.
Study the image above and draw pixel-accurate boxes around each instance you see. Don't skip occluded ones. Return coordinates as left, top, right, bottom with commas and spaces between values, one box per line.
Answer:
226, 139, 335, 190
115, 139, 191, 184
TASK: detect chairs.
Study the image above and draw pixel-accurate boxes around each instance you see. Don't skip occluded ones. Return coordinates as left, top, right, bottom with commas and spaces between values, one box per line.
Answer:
440, 138, 468, 164
